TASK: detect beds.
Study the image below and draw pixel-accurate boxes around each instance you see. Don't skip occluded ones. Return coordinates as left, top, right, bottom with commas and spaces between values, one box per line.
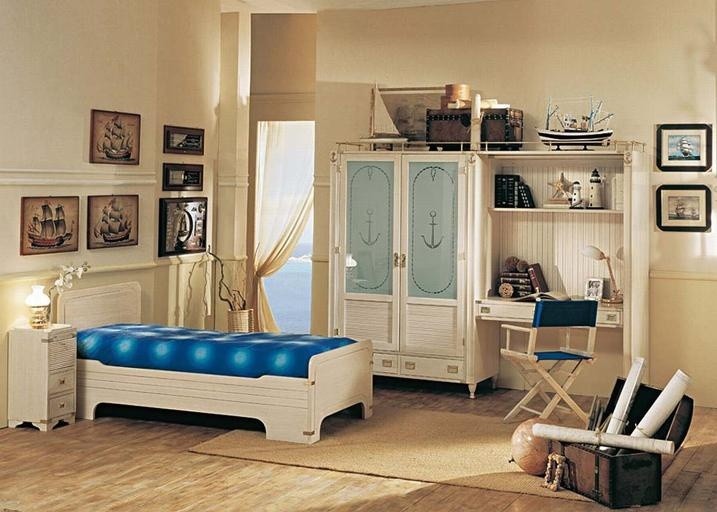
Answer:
51, 280, 376, 443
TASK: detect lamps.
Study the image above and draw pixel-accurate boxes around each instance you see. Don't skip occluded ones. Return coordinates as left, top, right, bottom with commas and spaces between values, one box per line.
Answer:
582, 245, 622, 303
29, 284, 50, 329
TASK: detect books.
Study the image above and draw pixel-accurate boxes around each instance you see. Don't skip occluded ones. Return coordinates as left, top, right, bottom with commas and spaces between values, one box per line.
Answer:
495, 174, 536, 208
497, 262, 570, 302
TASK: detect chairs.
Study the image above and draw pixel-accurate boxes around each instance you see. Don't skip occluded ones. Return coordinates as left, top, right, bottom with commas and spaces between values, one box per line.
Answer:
499, 297, 598, 423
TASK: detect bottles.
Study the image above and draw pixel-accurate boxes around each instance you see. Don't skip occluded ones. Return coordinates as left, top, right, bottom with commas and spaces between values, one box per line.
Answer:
31, 310, 50, 331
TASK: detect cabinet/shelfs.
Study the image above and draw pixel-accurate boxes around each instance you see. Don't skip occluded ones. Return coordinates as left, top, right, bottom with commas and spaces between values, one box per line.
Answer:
474, 141, 652, 385
329, 138, 500, 400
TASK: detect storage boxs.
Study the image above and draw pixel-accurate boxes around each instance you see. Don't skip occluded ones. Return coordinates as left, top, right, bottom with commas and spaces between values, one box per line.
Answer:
426, 107, 524, 151
552, 376, 694, 508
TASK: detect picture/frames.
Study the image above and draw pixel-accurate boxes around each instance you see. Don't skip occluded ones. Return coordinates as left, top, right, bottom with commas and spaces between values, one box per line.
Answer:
656, 183, 712, 232
163, 125, 205, 156
656, 123, 712, 172
163, 163, 203, 191
584, 278, 604, 302
159, 197, 207, 257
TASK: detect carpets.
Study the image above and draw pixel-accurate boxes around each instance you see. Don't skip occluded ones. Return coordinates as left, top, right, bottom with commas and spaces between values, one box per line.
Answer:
188, 406, 596, 503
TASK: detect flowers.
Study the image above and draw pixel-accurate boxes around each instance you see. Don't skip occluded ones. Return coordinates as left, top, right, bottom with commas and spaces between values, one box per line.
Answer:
48, 260, 91, 294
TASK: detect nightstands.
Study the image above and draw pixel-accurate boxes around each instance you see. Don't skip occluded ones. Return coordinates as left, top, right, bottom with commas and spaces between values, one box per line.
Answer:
7, 323, 77, 432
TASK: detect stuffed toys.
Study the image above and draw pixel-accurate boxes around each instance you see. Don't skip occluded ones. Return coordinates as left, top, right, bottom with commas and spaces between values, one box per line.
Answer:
505, 255, 529, 272
